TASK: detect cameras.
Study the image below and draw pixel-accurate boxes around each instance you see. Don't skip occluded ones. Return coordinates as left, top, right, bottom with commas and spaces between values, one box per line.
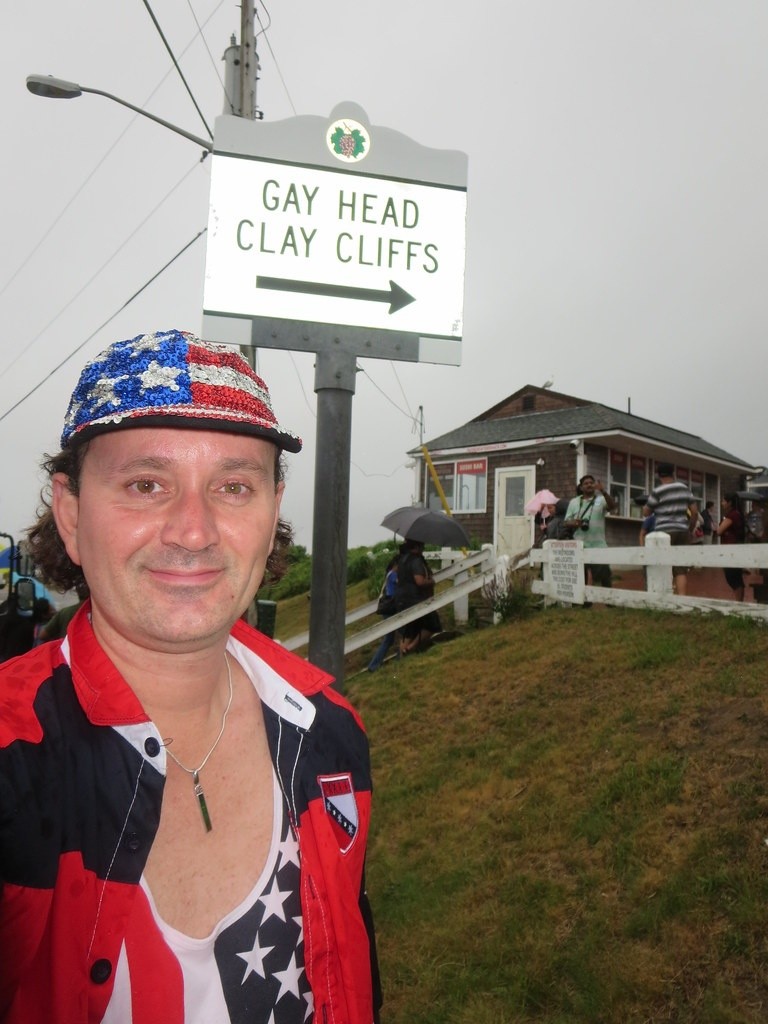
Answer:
578, 518, 589, 531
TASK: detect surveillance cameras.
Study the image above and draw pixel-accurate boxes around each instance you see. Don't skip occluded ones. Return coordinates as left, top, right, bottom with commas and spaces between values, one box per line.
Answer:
570, 440, 580, 449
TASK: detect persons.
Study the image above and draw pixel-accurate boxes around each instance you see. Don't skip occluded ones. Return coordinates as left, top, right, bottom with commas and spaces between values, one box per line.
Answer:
700, 501, 717, 544
716, 491, 747, 601
686, 493, 705, 545
0, 330, 383, 1024
369, 538, 444, 672
562, 475, 618, 608
535, 501, 575, 610
746, 501, 765, 543
639, 463, 698, 596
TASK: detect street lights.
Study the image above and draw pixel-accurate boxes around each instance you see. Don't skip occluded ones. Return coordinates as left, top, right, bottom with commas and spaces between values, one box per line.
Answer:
20, 73, 260, 365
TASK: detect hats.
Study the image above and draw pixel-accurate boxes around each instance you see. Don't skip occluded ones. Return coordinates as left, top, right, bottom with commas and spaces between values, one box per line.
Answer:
658, 463, 675, 472
60, 328, 303, 455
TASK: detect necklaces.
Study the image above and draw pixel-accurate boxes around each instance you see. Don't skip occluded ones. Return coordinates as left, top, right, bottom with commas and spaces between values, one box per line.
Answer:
165, 650, 232, 831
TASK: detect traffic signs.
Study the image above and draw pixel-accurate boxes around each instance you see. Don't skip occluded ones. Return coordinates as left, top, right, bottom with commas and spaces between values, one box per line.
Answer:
204, 96, 468, 371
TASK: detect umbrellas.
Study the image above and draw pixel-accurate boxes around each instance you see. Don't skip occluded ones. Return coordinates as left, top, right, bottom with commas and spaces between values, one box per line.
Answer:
383, 507, 472, 547
526, 490, 561, 526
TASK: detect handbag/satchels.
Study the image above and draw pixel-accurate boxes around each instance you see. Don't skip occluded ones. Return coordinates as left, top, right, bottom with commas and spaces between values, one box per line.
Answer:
377, 585, 397, 615
